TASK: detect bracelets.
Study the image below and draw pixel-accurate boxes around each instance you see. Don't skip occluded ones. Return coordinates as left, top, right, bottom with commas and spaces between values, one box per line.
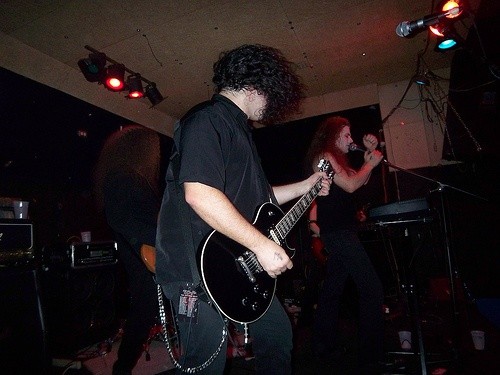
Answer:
308, 220, 317, 223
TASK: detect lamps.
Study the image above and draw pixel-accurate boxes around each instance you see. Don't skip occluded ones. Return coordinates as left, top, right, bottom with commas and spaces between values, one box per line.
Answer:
144, 82, 169, 109
125, 72, 144, 99
78, 53, 109, 83
102, 62, 127, 92
428, 0, 467, 52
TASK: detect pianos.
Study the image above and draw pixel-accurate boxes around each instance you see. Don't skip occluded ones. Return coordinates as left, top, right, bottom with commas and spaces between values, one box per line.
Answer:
360, 196, 441, 316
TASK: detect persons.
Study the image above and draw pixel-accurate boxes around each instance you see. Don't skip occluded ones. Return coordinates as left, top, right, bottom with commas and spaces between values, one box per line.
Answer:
102, 129, 165, 375
155, 45, 333, 375
307, 116, 406, 375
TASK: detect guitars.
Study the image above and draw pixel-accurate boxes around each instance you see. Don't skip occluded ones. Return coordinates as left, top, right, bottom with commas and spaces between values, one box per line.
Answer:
196, 158, 337, 324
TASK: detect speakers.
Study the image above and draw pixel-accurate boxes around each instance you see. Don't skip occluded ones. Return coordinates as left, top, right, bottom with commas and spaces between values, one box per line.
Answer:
52, 265, 118, 350
0, 270, 51, 375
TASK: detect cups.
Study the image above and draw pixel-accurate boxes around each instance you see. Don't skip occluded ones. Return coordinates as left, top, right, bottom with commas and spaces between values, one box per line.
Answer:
398, 331, 411, 349
12, 201, 29, 219
81, 232, 91, 242
471, 331, 484, 350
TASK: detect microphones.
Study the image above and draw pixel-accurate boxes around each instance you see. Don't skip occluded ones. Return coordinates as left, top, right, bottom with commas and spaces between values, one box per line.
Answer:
396, 11, 450, 37
350, 143, 372, 154
